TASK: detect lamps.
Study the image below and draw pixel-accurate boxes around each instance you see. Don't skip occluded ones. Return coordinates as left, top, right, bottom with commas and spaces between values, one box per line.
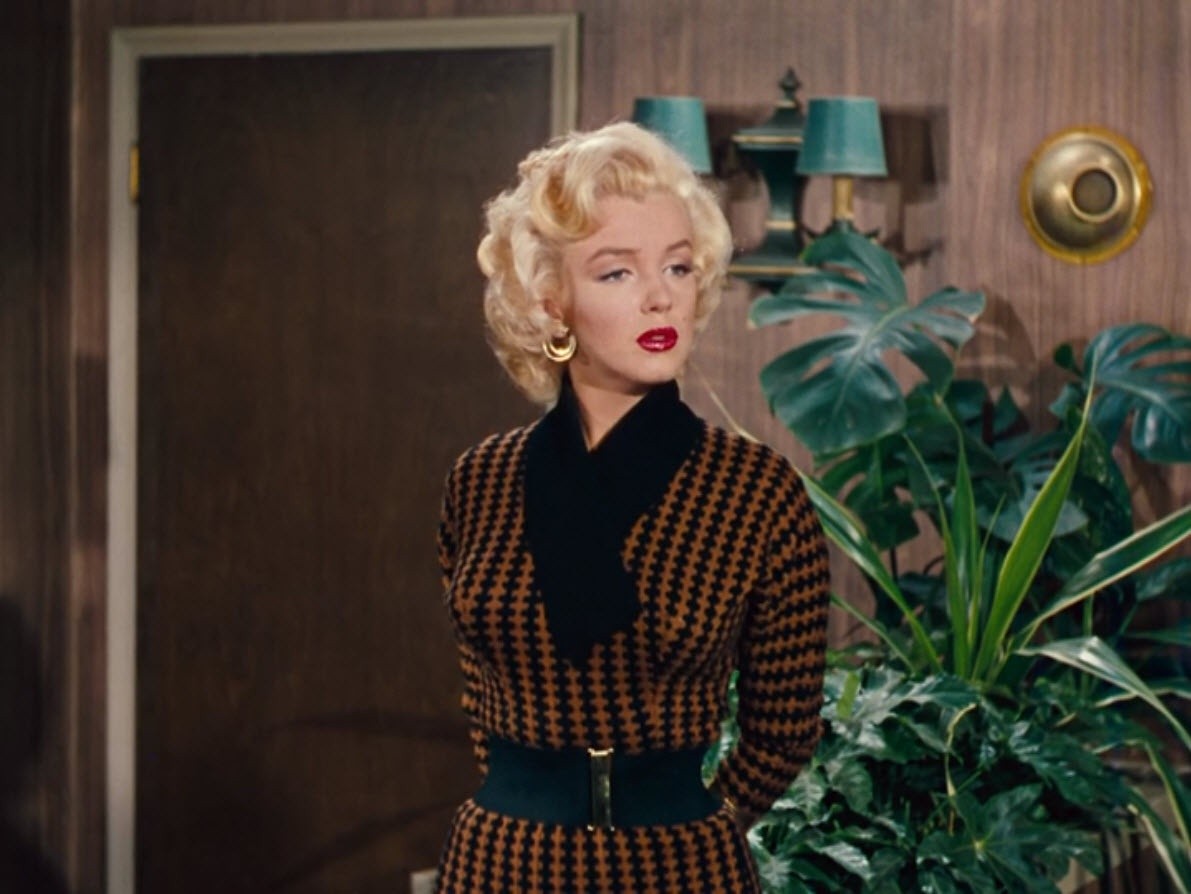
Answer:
634, 66, 892, 292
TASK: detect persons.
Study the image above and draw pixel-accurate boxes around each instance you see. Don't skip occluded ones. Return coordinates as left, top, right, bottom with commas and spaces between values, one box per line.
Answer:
433, 123, 831, 894
